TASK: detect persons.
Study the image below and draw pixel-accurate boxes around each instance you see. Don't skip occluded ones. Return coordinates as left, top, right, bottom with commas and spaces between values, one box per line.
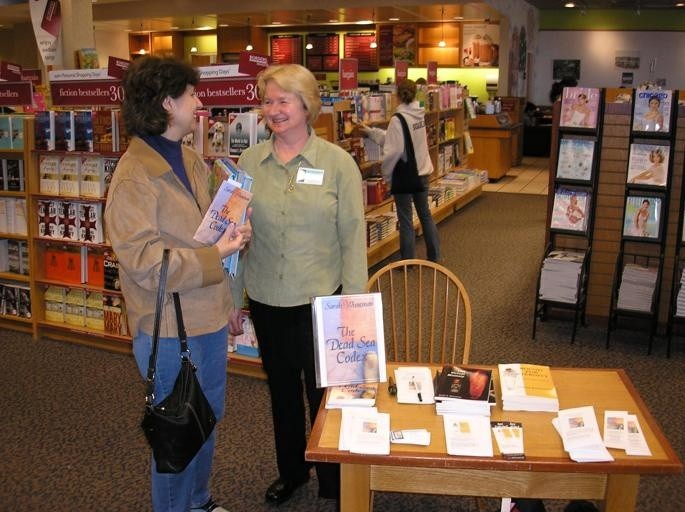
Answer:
565, 194, 588, 224
568, 94, 590, 127
629, 149, 666, 186
522, 101, 537, 129
230, 63, 370, 512
633, 200, 651, 237
102, 50, 252, 512
549, 75, 580, 103
635, 96, 665, 132
353, 79, 443, 269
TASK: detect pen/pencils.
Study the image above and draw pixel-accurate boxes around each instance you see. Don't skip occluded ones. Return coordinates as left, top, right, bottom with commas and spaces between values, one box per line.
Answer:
412, 375, 423, 402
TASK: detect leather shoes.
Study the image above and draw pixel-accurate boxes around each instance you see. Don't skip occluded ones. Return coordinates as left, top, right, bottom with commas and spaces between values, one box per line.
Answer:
265, 477, 310, 503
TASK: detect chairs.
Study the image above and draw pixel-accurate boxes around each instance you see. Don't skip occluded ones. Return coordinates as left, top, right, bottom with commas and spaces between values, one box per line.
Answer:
360, 258, 470, 368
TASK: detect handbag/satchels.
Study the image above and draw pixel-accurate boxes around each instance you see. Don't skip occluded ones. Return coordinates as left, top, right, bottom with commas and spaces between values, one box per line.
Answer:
141, 355, 217, 472
391, 158, 426, 193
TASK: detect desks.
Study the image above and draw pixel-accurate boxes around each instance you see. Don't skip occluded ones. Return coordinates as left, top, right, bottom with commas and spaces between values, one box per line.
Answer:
306, 361, 683, 512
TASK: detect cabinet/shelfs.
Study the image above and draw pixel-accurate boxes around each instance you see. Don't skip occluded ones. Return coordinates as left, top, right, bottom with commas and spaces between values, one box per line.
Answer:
128, 25, 252, 66
374, 18, 501, 68
0, 86, 132, 353
532, 86, 607, 347
606, 86, 680, 356
310, 86, 485, 270
183, 89, 267, 380
665, 153, 685, 358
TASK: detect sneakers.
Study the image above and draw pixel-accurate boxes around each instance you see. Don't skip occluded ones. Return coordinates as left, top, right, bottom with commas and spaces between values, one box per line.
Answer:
204, 497, 225, 511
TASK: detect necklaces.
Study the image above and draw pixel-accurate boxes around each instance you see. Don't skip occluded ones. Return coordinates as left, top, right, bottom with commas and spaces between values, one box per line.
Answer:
287, 160, 303, 192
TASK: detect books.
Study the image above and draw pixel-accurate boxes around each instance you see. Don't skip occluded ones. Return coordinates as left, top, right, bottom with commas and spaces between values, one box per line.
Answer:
633, 89, 672, 133
557, 138, 594, 181
1, 197, 105, 243
540, 249, 586, 306
1, 286, 131, 337
2, 238, 121, 291
627, 141, 671, 187
549, 186, 591, 233
1, 100, 127, 155
675, 267, 685, 318
229, 310, 261, 356
184, 107, 272, 155
318, 83, 487, 251
625, 196, 662, 238
2, 157, 122, 198
309, 292, 387, 388
191, 157, 254, 275
324, 363, 559, 460
616, 263, 659, 313
559, 87, 601, 128
